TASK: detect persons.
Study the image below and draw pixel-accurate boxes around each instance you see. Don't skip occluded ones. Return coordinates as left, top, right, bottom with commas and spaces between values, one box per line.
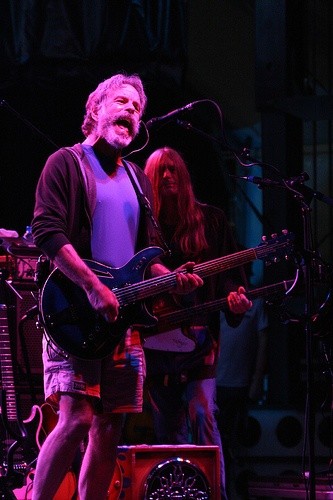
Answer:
216, 269, 271, 500
30, 74, 204, 500
143, 146, 253, 500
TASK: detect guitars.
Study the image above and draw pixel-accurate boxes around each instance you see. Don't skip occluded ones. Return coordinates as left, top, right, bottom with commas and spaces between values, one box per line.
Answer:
0, 303, 45, 500
138, 277, 297, 353
40, 402, 123, 500
37, 228, 292, 362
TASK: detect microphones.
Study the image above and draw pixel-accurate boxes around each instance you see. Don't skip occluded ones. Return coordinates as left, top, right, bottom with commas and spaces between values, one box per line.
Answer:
146, 100, 199, 132
240, 174, 285, 192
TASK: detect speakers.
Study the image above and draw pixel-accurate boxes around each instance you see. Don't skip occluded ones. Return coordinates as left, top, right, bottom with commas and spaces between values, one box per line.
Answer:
5, 287, 43, 388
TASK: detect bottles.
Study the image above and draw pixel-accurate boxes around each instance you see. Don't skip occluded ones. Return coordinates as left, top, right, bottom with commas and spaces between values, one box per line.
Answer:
22, 226, 34, 244
